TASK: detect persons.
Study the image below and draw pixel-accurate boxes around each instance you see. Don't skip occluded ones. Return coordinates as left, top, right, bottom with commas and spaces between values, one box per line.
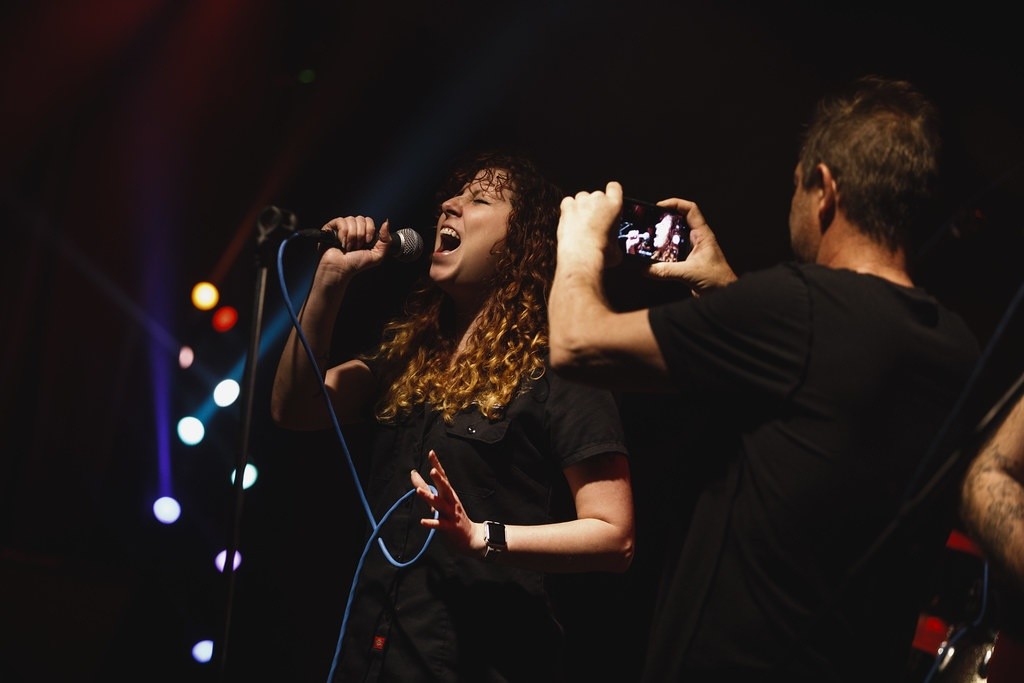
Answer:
270, 151, 636, 682
547, 75, 988, 682
961, 390, 1024, 581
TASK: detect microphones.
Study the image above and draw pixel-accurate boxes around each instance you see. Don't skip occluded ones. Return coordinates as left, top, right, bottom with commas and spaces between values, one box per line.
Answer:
302, 227, 424, 264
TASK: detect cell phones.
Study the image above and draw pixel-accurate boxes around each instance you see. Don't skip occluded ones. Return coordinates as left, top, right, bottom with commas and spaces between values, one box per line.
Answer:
617, 197, 689, 263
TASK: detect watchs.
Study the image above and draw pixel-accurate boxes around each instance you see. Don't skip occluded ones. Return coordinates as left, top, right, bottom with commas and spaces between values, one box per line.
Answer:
483, 520, 506, 563
